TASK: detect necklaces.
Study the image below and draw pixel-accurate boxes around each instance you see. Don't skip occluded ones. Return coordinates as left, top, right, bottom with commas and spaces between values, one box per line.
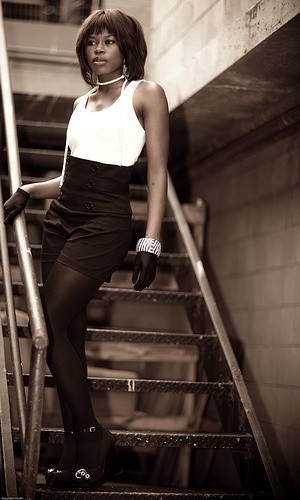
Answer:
95, 74, 126, 86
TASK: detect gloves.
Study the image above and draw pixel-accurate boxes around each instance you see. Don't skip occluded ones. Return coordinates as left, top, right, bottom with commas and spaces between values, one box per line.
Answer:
3, 188, 30, 224
132, 251, 157, 291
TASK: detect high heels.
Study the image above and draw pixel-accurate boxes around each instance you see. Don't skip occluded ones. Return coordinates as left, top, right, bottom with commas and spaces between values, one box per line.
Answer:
46, 431, 77, 490
70, 425, 123, 491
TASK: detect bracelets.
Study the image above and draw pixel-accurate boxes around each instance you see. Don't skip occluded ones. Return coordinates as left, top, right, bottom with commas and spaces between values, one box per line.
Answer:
135, 237, 162, 258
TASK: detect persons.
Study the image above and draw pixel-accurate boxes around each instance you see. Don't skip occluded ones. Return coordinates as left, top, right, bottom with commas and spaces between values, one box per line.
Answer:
2, 7, 170, 493
